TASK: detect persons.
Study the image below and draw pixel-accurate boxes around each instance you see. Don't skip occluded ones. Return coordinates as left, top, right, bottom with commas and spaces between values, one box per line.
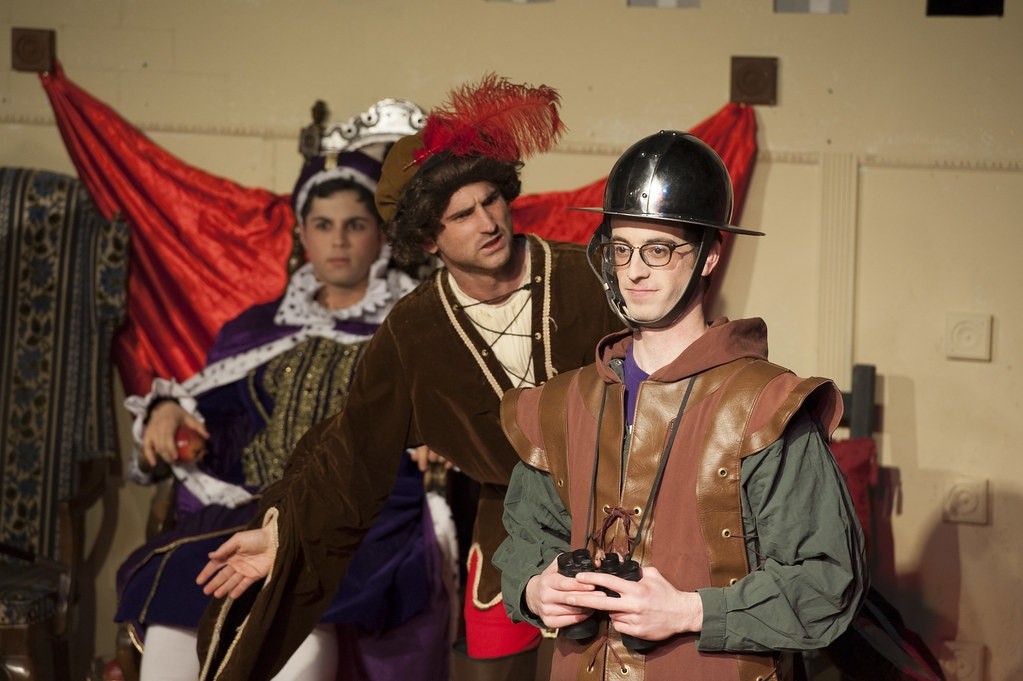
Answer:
113, 97, 460, 681
492, 130, 864, 680
195, 74, 626, 680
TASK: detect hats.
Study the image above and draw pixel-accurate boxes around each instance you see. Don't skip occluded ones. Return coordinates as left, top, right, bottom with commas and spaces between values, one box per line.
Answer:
293, 152, 383, 226
373, 69, 569, 226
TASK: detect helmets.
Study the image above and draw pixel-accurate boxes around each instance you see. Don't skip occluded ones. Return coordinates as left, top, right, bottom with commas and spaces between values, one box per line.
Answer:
566, 130, 766, 238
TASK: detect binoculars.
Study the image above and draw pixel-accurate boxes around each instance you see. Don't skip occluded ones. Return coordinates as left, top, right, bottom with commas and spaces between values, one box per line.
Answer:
556, 549, 657, 651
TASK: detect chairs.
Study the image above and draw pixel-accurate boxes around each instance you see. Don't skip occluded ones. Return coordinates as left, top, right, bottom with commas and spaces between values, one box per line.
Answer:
0, 166, 129, 680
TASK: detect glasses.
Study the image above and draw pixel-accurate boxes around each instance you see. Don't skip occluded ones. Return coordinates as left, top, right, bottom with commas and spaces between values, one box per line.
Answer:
600, 239, 700, 267
